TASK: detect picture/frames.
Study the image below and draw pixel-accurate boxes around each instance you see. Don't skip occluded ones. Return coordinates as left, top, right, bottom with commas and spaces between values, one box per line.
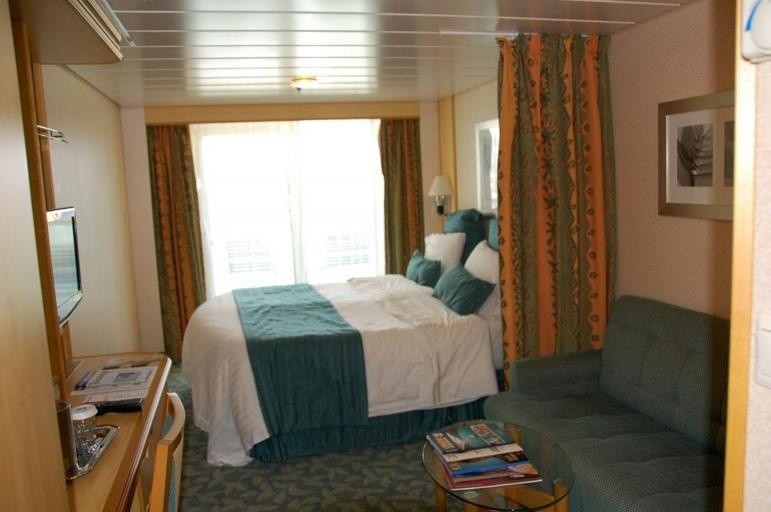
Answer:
658, 88, 734, 224
475, 118, 499, 215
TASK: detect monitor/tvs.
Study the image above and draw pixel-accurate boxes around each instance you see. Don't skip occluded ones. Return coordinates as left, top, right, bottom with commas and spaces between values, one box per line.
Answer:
47, 207, 83, 326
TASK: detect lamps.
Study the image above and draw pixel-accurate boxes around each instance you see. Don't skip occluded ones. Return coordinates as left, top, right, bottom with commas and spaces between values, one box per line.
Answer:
427, 176, 452, 215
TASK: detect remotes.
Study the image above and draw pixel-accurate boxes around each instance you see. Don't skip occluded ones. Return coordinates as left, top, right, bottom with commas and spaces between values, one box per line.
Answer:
85, 398, 144, 415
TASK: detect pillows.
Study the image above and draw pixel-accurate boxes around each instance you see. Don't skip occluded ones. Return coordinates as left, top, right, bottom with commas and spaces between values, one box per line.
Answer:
405, 209, 504, 322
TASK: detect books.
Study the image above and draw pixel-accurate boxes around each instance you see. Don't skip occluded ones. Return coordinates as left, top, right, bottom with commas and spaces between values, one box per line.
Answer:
68, 353, 166, 404
426, 421, 545, 491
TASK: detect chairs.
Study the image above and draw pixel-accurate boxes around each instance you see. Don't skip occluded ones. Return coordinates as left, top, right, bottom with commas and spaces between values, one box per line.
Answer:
147, 392, 188, 512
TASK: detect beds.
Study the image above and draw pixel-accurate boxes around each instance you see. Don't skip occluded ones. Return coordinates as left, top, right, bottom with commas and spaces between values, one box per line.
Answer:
187, 210, 513, 464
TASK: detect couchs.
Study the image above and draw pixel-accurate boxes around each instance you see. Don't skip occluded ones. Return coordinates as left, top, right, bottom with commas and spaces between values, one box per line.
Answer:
482, 295, 729, 512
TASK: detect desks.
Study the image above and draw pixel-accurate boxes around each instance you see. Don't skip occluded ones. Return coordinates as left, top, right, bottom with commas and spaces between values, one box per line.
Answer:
64, 353, 172, 512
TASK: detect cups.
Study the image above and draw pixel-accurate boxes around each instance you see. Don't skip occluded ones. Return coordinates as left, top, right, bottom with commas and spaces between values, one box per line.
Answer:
71, 404, 98, 466
